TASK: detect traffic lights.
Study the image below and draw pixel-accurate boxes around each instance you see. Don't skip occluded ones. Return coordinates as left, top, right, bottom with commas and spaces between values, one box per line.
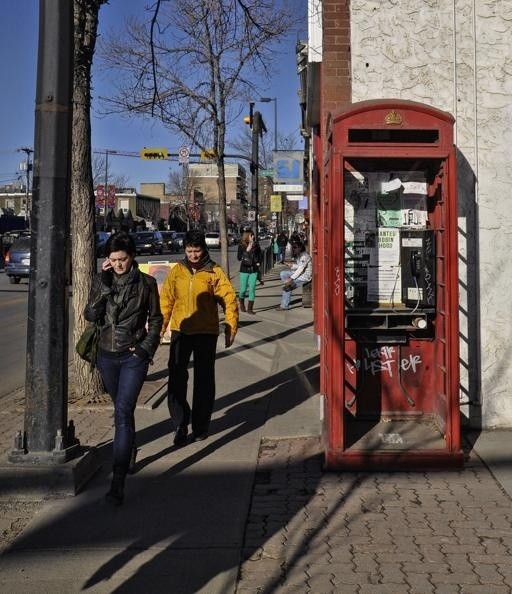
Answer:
140, 148, 168, 160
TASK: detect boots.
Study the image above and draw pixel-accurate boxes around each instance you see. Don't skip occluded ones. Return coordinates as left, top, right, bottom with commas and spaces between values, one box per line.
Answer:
238, 296, 246, 313
127, 443, 137, 475
247, 299, 256, 314
104, 464, 127, 505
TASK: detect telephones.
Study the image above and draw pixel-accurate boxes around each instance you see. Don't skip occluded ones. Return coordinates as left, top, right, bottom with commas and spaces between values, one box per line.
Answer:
410, 250, 423, 276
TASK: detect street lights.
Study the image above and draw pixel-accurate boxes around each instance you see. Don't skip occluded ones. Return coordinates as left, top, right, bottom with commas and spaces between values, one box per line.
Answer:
103, 149, 118, 231
259, 97, 278, 150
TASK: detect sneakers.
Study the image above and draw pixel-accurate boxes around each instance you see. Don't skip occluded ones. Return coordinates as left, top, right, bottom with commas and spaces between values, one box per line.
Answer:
173, 424, 188, 445
275, 305, 289, 311
187, 429, 208, 442
282, 281, 298, 291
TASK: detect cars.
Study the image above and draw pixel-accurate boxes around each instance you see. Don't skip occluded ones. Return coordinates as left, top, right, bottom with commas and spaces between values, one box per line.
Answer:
1, 227, 277, 286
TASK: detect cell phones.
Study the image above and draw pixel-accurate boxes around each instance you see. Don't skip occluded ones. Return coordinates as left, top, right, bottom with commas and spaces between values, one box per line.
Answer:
105, 257, 111, 271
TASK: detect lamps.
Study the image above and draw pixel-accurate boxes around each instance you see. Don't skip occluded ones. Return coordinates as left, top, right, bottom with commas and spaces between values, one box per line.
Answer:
295, 29, 309, 75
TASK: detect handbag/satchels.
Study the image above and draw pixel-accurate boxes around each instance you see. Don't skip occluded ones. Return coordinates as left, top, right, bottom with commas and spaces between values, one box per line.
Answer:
75, 314, 101, 368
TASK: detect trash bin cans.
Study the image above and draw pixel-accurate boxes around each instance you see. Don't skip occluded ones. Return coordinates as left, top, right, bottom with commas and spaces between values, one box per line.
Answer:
302, 282, 313, 308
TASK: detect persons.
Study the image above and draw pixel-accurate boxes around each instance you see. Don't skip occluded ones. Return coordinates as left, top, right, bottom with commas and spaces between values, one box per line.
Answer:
276, 241, 313, 311
160, 229, 238, 447
272, 230, 308, 263
237, 230, 262, 314
84, 231, 164, 505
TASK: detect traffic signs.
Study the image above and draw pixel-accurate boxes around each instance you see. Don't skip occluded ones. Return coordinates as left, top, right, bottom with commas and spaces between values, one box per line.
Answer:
258, 170, 278, 177
